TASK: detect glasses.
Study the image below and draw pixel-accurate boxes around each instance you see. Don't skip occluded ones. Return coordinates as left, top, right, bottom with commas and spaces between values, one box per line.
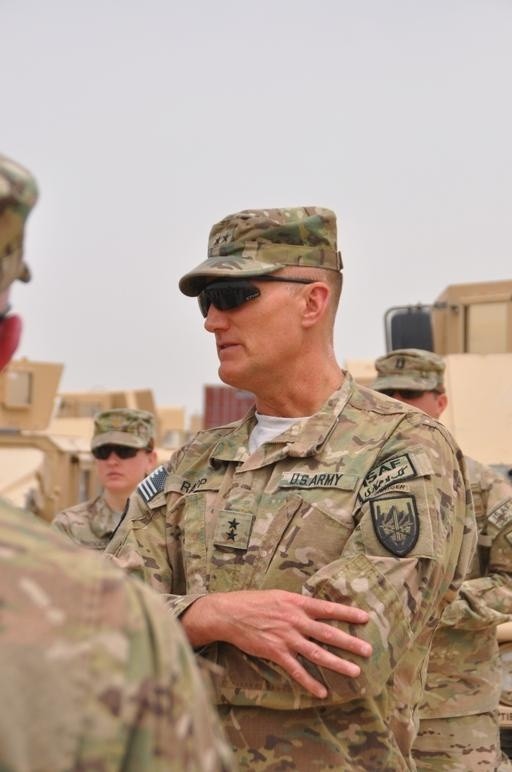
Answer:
198, 276, 316, 318
93, 445, 149, 459
379, 391, 426, 400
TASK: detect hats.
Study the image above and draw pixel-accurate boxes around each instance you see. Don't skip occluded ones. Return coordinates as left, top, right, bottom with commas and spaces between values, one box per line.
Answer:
177, 206, 343, 298
372, 348, 446, 393
0, 154, 38, 293
91, 408, 155, 453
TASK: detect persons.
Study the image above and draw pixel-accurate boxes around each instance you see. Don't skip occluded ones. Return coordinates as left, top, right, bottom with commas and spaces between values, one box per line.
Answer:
50, 407, 157, 555
0, 153, 240, 772
101, 206, 477, 772
371, 348, 512, 772
